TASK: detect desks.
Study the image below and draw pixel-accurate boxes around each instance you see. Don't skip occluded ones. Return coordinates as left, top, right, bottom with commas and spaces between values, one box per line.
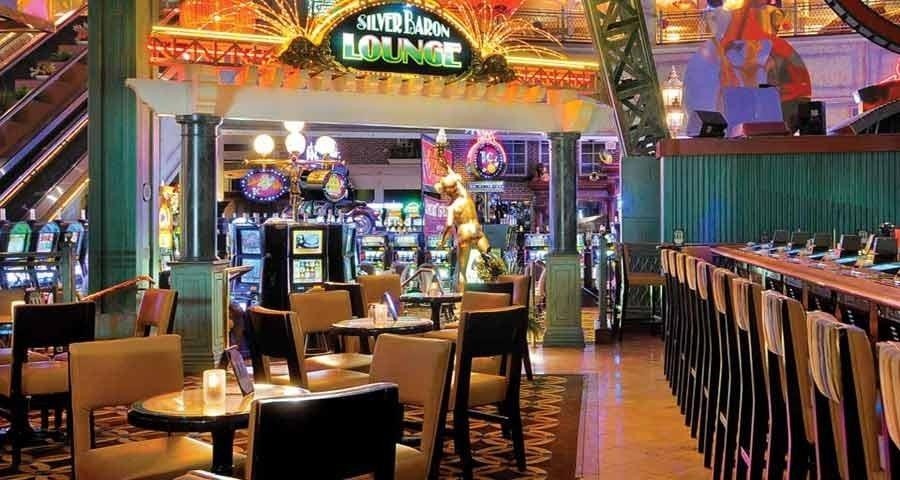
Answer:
0, 314, 40, 445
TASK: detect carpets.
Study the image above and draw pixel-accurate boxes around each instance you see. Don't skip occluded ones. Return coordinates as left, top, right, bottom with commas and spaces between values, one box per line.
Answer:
0, 371, 602, 480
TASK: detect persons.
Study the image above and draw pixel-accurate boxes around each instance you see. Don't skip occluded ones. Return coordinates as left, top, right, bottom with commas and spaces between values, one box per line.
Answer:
435, 152, 491, 293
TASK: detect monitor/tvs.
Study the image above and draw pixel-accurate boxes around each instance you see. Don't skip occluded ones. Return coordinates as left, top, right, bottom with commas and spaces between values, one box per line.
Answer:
240, 256, 263, 285
342, 257, 354, 279
289, 227, 327, 258
4, 262, 32, 287
291, 258, 325, 285
33, 264, 66, 285
239, 227, 265, 257
345, 228, 356, 255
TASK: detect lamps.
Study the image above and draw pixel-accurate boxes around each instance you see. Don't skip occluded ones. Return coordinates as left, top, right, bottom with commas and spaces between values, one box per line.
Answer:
253, 134, 276, 158
315, 135, 337, 156
283, 121, 305, 133
284, 132, 306, 159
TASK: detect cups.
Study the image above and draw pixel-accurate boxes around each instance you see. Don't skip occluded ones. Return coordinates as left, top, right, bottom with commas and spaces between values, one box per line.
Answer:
203, 369, 226, 404
367, 303, 386, 322
10, 300, 25, 323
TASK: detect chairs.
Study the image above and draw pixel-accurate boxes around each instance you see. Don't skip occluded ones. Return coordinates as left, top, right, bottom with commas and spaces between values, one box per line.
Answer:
611, 244, 665, 342
0, 300, 96, 467
